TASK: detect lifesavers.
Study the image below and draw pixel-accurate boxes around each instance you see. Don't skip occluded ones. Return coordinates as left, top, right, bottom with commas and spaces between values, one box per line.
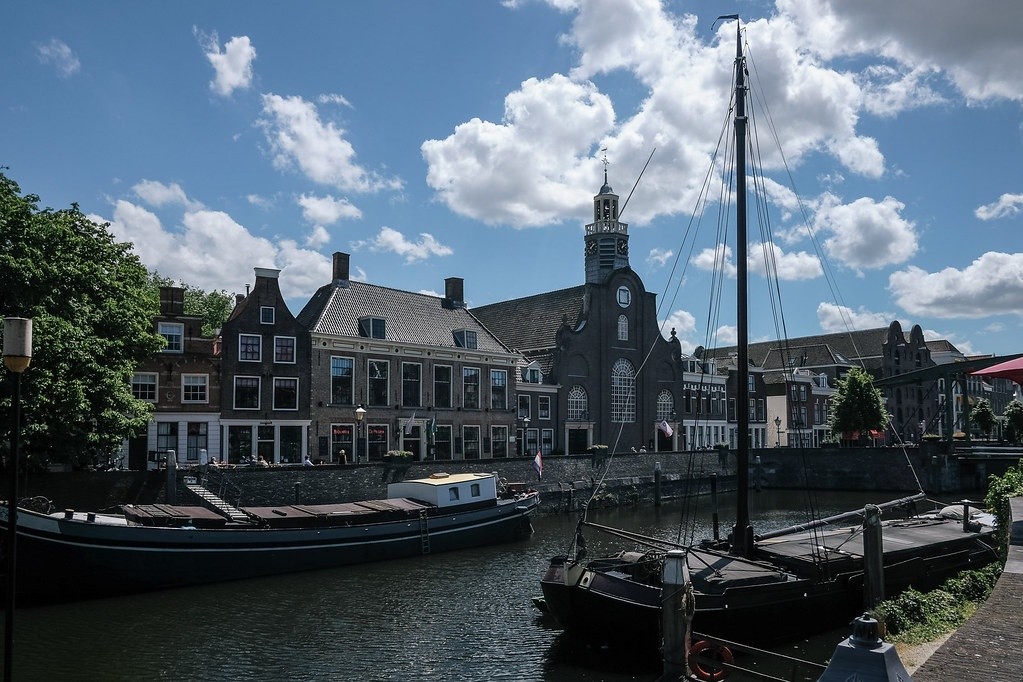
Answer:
429, 475, 445, 479
433, 473, 450, 477
688, 642, 734, 681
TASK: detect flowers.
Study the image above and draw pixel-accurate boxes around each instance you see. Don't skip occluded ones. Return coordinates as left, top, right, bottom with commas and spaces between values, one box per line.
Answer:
384, 450, 414, 458
587, 444, 608, 450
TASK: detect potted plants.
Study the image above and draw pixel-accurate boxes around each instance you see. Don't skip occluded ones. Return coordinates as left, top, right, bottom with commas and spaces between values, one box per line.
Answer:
714, 440, 730, 457
819, 437, 840, 448
923, 434, 943, 448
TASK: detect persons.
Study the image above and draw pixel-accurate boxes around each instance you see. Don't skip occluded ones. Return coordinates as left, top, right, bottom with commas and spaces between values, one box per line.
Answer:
209, 457, 217, 464
639, 446, 646, 452
241, 455, 268, 465
304, 455, 313, 466
631, 447, 637, 453
338, 450, 347, 464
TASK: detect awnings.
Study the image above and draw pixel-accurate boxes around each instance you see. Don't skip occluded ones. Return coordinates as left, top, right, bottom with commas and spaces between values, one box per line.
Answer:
845, 430, 885, 439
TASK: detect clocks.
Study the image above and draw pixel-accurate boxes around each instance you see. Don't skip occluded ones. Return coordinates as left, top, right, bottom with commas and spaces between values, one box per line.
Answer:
586, 239, 598, 256
616, 238, 628, 256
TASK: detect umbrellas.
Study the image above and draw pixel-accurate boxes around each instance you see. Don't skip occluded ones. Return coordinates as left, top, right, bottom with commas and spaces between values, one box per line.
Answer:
970, 357, 1023, 390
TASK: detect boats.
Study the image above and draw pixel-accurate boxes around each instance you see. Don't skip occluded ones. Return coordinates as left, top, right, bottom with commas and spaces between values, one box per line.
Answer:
0, 465, 545, 588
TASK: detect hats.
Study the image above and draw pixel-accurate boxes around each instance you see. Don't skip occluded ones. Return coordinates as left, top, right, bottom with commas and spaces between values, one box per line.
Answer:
339, 450, 345, 454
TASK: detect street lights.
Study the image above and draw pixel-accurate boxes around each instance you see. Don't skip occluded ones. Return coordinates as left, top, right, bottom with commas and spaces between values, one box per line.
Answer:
0, 317, 35, 682
353, 403, 366, 466
886, 412, 896, 447
519, 414, 532, 457
773, 417, 782, 449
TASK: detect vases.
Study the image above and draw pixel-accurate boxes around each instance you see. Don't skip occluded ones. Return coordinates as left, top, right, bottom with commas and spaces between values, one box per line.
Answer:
590, 448, 609, 458
383, 456, 413, 468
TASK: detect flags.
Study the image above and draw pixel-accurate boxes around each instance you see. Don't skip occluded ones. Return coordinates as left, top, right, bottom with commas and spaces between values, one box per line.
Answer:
660, 421, 672, 437
427, 413, 437, 436
532, 450, 542, 480
405, 412, 415, 434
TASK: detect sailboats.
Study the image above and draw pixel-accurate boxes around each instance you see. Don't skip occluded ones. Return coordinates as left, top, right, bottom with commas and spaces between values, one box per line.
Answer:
529, 10, 1006, 644
